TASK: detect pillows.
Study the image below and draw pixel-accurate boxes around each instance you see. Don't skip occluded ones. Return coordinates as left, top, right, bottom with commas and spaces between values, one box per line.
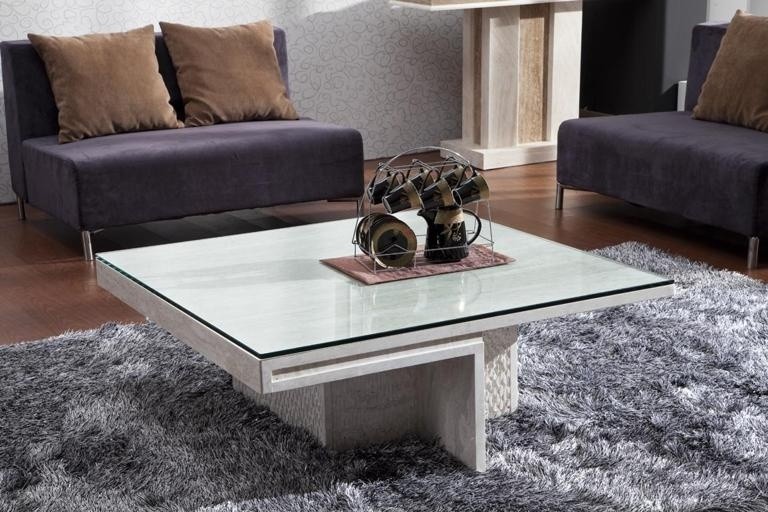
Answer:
691, 7, 768, 131
23, 24, 185, 144
158, 19, 299, 129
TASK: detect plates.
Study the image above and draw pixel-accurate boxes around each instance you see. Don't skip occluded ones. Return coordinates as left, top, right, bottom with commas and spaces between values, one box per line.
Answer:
356, 213, 417, 270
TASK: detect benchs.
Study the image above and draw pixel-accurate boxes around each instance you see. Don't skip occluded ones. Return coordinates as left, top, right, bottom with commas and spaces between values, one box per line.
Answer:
0, 24, 371, 261
556, 21, 768, 272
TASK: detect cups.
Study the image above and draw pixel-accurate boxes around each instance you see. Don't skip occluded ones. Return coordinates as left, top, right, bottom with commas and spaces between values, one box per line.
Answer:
367, 156, 489, 214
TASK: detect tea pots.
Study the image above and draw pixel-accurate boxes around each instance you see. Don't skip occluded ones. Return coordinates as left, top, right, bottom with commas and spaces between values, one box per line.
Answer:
417, 205, 481, 263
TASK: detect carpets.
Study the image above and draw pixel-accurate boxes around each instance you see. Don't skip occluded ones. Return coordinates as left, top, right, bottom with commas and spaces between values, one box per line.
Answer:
0, 239, 767, 511
